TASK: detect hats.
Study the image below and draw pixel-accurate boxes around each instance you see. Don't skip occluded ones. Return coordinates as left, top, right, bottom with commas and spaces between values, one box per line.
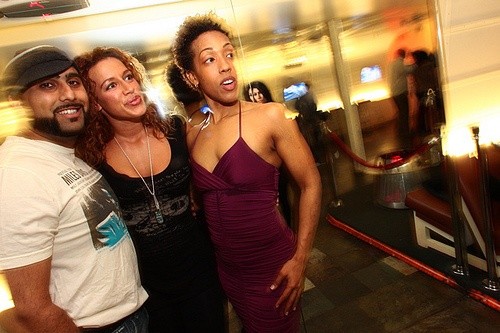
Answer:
2, 44, 75, 100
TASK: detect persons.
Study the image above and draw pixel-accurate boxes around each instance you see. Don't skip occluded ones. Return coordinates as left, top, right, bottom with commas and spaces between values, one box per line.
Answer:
165, 15, 447, 333
72, 46, 230, 333
0, 44, 150, 333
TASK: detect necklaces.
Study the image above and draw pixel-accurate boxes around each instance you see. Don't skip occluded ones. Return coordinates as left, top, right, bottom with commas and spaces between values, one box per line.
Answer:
114, 124, 164, 224
